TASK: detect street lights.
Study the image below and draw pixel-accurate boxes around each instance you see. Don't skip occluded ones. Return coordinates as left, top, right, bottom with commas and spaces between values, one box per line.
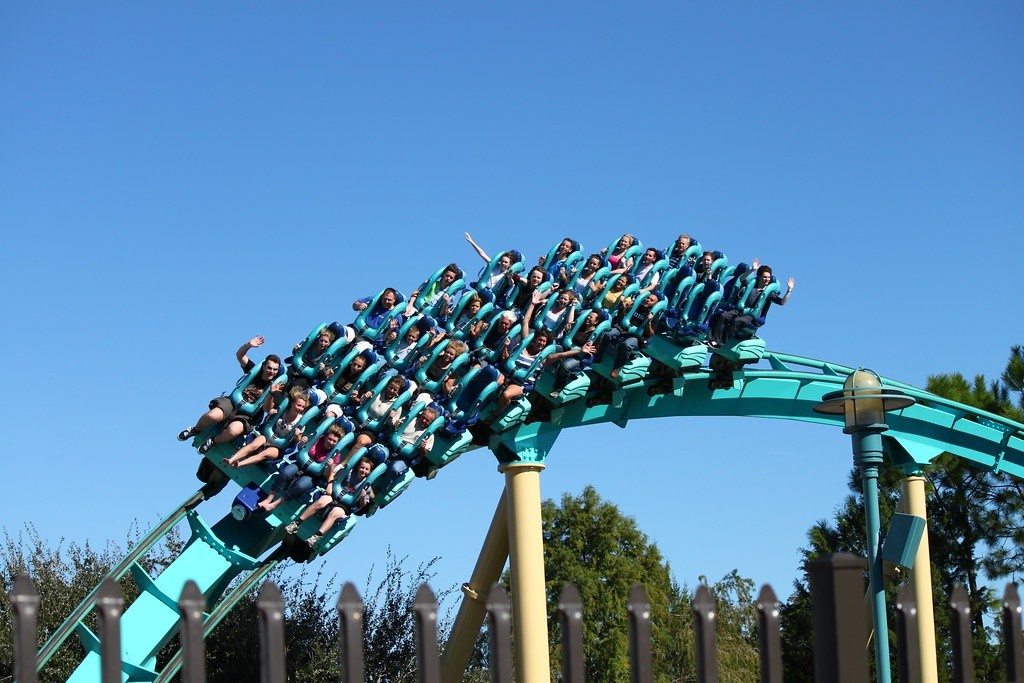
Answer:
814, 367, 926, 683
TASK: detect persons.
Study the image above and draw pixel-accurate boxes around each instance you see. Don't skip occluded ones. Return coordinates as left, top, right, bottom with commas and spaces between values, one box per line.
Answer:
177, 232, 795, 548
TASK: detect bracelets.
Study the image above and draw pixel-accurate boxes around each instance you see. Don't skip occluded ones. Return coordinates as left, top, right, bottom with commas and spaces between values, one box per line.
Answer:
572, 305, 577, 306
788, 289, 792, 292
268, 390, 274, 396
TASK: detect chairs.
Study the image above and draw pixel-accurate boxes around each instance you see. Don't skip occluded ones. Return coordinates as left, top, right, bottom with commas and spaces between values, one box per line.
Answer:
195, 233, 784, 541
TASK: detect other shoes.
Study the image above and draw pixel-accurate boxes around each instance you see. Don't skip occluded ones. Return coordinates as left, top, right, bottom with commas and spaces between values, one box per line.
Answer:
196, 435, 215, 456
306, 530, 324, 548
176, 426, 201, 440
285, 519, 300, 535
612, 367, 620, 380
698, 338, 723, 350
549, 390, 563, 399
